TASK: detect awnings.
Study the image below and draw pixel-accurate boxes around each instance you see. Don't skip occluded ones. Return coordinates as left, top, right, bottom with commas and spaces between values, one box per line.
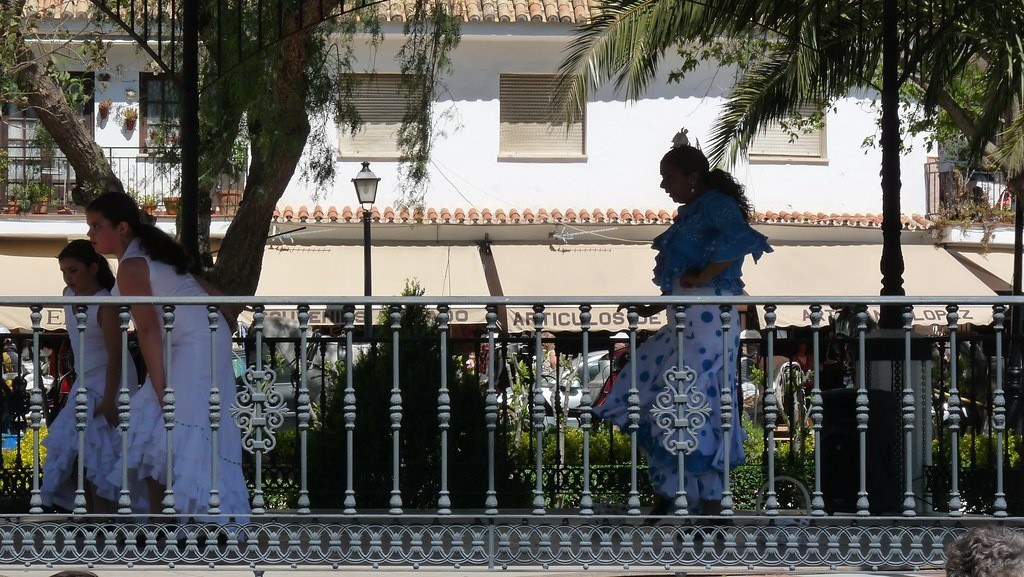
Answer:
948, 246, 1024, 292
0, 241, 1000, 331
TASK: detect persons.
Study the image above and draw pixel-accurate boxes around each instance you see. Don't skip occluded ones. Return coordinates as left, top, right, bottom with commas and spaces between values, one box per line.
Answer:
945, 527, 1024, 577
972, 186, 988, 205
609, 331, 629, 351
0, 327, 18, 389
792, 340, 823, 389
40, 239, 152, 537
85, 191, 253, 546
541, 332, 557, 369
592, 146, 774, 542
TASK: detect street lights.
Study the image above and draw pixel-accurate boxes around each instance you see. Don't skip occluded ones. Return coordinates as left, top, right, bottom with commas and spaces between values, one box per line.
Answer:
349, 161, 382, 337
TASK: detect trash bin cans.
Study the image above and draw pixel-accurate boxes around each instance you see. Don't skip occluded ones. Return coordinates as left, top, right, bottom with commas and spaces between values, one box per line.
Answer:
810, 388, 905, 515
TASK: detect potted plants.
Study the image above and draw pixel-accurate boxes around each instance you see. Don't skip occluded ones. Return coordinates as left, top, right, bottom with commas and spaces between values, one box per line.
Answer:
145, 128, 181, 153
125, 89, 136, 100
123, 107, 138, 130
163, 196, 178, 214
5, 185, 28, 214
28, 183, 57, 214
35, 126, 53, 157
141, 195, 155, 215
97, 73, 111, 91
99, 99, 112, 118
215, 139, 248, 214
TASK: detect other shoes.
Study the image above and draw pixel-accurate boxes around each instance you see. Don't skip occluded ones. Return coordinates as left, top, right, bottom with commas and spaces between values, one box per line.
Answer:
643, 502, 668, 526
676, 512, 729, 543
173, 526, 231, 547
62, 519, 116, 539
120, 521, 181, 547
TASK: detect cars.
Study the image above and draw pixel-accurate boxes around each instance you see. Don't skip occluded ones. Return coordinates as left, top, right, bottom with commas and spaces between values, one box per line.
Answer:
495, 350, 610, 432
233, 349, 300, 404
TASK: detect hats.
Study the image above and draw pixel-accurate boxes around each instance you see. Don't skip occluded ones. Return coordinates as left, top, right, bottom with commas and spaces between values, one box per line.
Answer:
608, 332, 629, 339
